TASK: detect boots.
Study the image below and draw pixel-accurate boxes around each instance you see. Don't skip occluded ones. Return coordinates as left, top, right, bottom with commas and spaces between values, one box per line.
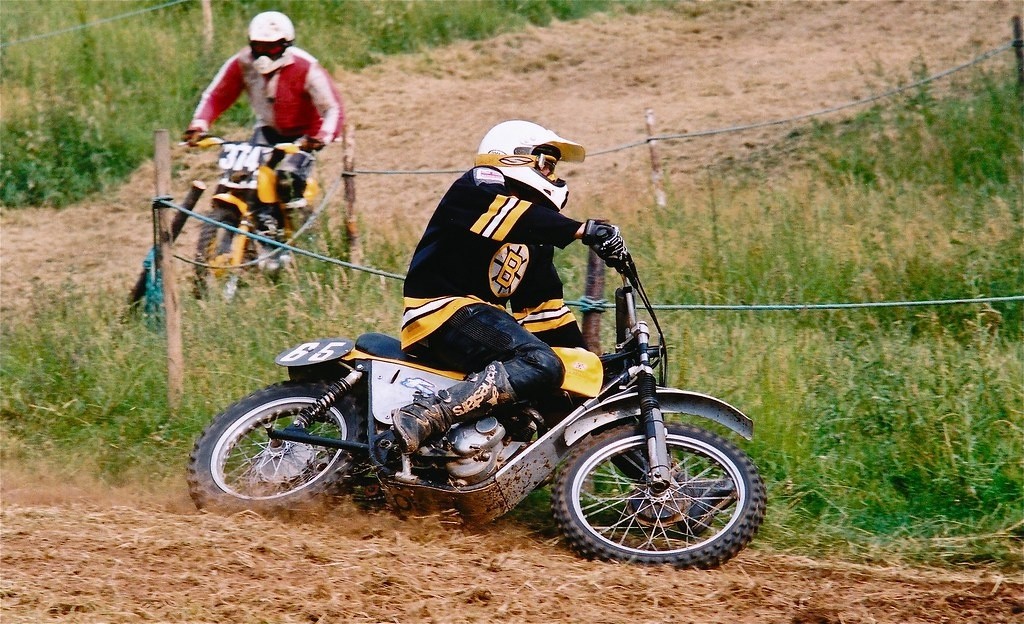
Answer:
610, 447, 737, 537
280, 198, 310, 232
391, 360, 518, 457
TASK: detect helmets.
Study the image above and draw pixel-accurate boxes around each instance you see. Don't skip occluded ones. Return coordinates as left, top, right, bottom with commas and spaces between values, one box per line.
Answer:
473, 120, 585, 210
248, 11, 296, 74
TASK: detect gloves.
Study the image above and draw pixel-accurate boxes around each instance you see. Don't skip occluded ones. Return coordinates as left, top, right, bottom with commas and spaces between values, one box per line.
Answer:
293, 134, 326, 152
582, 219, 628, 261
182, 124, 202, 147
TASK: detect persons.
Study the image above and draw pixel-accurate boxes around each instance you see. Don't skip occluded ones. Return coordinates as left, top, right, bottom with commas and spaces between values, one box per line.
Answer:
392, 120, 735, 531
181, 10, 344, 278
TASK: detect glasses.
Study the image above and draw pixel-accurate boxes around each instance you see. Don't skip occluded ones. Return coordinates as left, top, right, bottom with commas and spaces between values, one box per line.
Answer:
538, 155, 555, 175
250, 38, 286, 61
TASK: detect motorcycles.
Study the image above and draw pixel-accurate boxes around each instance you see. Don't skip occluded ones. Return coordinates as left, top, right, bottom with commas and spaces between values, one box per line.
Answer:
185, 220, 767, 571
180, 127, 327, 302
